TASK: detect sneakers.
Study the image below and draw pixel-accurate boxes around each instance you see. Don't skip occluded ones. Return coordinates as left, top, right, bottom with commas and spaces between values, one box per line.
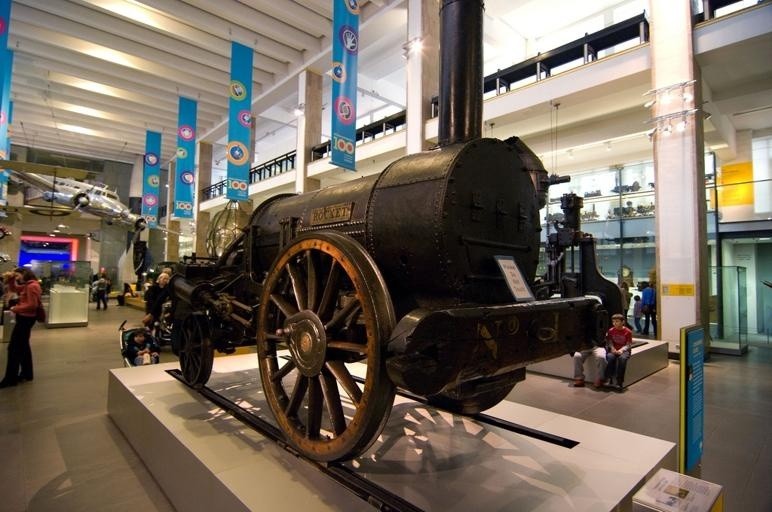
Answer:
572, 379, 626, 389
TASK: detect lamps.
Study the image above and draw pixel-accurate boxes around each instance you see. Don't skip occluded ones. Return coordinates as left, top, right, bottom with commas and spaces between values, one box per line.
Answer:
641, 78, 711, 141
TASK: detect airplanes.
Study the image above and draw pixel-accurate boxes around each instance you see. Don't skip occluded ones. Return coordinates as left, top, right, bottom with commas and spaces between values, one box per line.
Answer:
4, 170, 180, 236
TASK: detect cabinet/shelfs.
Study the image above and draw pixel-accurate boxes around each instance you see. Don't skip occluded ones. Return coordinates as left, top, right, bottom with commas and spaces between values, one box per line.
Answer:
538, 173, 715, 222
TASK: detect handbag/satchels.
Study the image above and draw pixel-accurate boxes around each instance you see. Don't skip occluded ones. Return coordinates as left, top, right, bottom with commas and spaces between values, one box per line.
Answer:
34, 295, 47, 324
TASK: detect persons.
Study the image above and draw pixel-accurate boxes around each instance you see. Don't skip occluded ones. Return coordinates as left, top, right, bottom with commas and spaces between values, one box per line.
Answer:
0, 267, 112, 388
572, 281, 657, 393
115, 267, 174, 366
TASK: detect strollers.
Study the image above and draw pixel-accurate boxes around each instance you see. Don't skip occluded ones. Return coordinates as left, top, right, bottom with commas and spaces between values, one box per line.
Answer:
117, 320, 162, 367
154, 303, 174, 347
